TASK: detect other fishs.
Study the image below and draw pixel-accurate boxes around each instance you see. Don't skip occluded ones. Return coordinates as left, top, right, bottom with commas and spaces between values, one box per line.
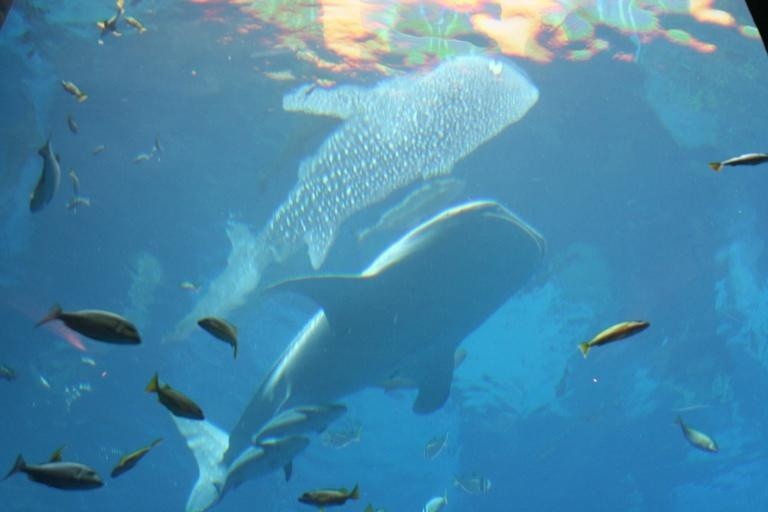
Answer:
4, 0, 548, 512
